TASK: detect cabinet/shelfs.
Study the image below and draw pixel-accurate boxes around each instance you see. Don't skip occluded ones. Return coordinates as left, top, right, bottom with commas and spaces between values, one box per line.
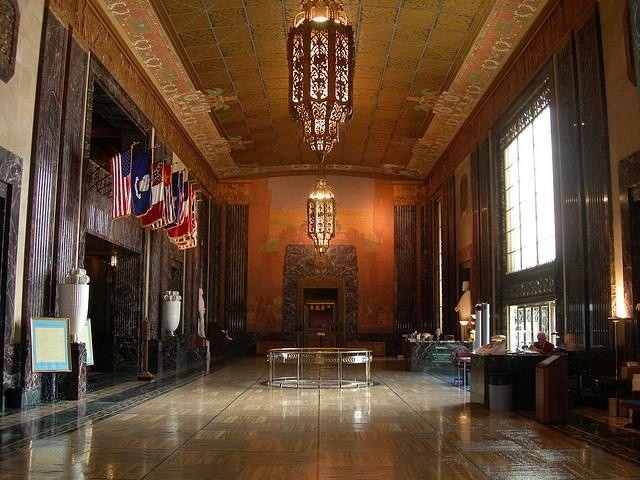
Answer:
256, 340, 297, 361
346, 340, 387, 358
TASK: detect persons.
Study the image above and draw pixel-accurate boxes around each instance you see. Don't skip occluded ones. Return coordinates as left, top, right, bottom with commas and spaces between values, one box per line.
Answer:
454, 281, 472, 343
529, 333, 554, 353
197, 288, 207, 339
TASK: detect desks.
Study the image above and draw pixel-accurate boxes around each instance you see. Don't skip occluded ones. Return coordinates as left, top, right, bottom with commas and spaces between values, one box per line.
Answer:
455, 357, 471, 387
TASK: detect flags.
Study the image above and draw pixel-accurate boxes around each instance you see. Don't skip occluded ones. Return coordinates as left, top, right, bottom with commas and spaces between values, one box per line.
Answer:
109, 144, 199, 250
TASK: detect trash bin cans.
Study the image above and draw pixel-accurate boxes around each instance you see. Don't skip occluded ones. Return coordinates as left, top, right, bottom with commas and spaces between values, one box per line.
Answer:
537, 354, 570, 424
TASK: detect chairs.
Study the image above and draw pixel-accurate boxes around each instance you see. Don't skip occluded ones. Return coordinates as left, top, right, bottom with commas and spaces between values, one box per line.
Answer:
454, 352, 473, 382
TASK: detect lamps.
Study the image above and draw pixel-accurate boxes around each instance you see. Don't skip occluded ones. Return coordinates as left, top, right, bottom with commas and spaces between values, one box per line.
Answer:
307, 171, 337, 260
285, 0, 356, 174
607, 316, 621, 379
459, 319, 470, 342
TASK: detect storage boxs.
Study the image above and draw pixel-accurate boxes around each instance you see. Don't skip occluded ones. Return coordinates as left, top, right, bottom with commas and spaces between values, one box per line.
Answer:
609, 362, 640, 417
